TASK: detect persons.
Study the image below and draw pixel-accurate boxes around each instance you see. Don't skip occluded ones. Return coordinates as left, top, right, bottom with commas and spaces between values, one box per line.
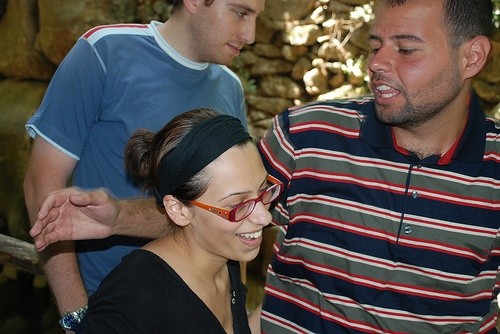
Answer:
28, 0, 500, 333
78, 108, 285, 334
24, 0, 268, 333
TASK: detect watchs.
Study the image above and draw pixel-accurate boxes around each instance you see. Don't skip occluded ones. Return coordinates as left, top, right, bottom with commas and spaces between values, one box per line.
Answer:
59, 303, 90, 332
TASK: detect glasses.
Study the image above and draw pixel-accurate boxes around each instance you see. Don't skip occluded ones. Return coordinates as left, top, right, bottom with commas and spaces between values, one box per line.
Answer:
177, 172, 284, 223
